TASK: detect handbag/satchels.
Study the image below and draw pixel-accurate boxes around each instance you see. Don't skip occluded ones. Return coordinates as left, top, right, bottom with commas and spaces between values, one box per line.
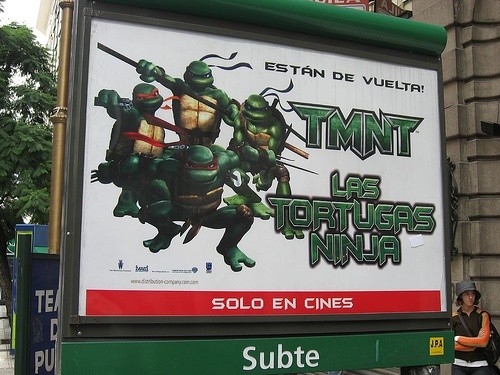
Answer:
474, 311, 499, 366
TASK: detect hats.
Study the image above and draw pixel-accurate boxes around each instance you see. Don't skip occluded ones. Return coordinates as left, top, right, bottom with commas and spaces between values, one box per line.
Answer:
456, 280, 481, 302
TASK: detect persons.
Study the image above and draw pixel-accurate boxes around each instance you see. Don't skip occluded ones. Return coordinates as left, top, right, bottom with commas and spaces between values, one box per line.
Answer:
452, 278, 492, 375
